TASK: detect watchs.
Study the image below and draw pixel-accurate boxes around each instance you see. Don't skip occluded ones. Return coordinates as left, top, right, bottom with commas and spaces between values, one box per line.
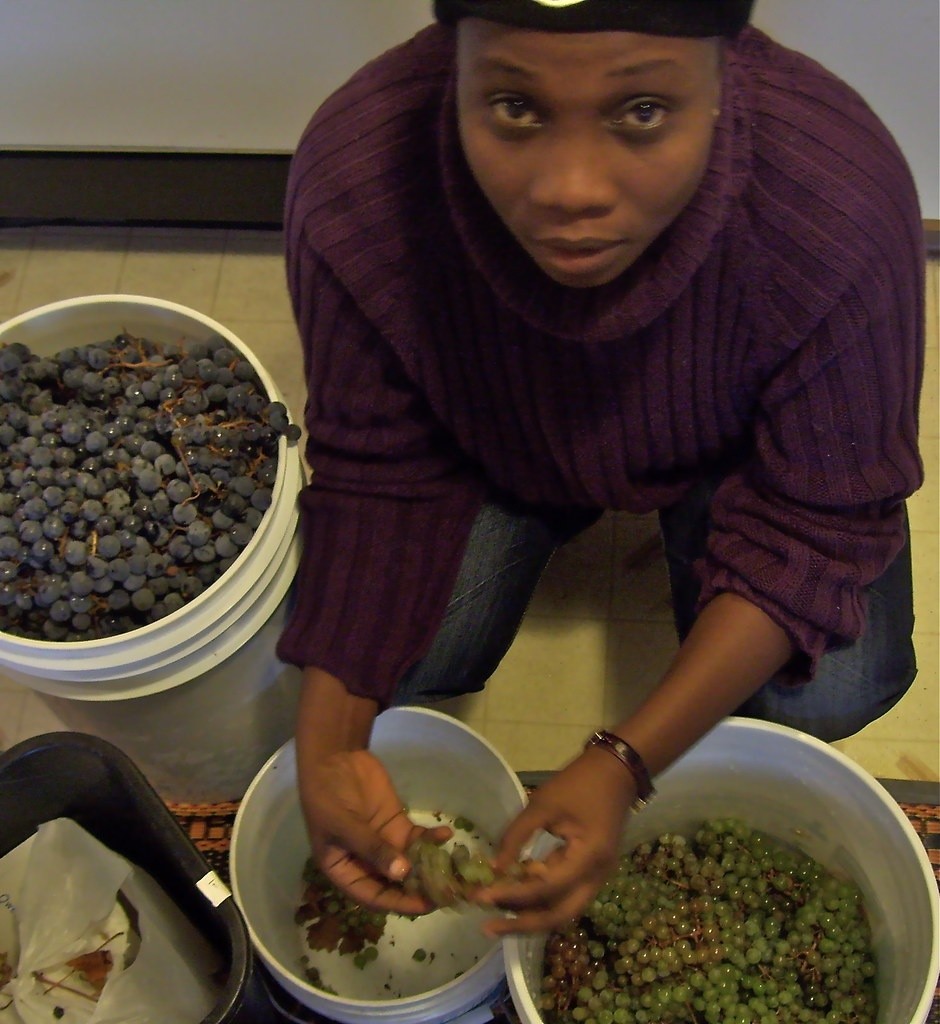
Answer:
586, 730, 657, 812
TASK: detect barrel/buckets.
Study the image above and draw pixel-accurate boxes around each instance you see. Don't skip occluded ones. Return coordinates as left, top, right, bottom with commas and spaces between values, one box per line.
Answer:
0, 294, 308, 804
228, 707, 530, 1023
501, 716, 940, 1024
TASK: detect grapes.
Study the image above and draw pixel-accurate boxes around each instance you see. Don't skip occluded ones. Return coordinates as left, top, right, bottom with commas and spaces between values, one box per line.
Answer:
0, 327, 302, 642
293, 802, 524, 996
539, 818, 879, 1024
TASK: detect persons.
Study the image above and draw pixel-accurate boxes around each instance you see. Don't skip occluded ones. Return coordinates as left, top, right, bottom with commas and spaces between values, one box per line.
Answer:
272, 0, 926, 937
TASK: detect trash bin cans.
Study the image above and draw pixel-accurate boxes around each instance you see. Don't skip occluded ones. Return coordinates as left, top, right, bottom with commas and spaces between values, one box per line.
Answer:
0, 731, 254, 1024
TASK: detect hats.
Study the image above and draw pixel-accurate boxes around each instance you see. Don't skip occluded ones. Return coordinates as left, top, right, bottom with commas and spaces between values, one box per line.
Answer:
434, 0, 753, 39
